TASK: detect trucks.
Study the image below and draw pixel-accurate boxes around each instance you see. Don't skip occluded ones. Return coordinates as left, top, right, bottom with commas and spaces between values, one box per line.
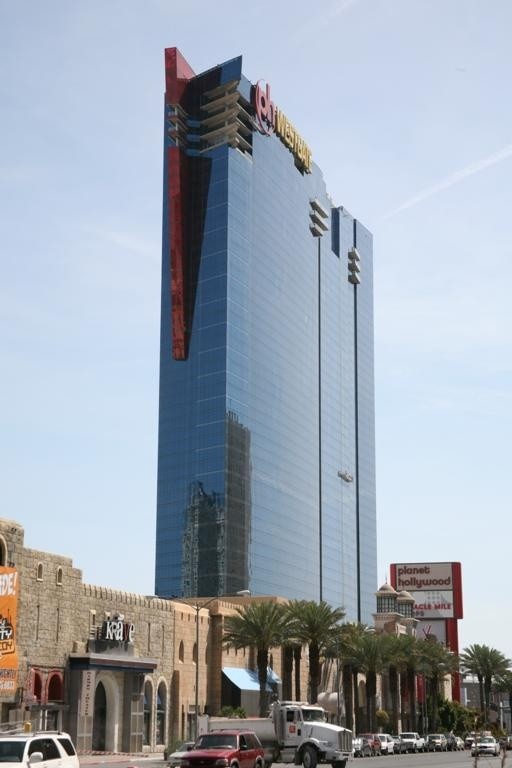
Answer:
196, 701, 356, 768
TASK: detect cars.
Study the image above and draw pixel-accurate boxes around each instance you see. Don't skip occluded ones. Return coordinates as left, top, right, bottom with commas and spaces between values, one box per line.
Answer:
167, 728, 265, 768
352, 733, 401, 757
424, 731, 512, 757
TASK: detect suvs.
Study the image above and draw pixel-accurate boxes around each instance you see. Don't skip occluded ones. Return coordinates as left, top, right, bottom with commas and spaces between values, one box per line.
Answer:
0, 730, 79, 768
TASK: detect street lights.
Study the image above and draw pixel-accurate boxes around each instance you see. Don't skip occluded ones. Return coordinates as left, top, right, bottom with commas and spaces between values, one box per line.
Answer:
195, 589, 250, 742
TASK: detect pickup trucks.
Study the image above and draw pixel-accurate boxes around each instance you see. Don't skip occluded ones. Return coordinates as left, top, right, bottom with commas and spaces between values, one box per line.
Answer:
397, 732, 425, 754
